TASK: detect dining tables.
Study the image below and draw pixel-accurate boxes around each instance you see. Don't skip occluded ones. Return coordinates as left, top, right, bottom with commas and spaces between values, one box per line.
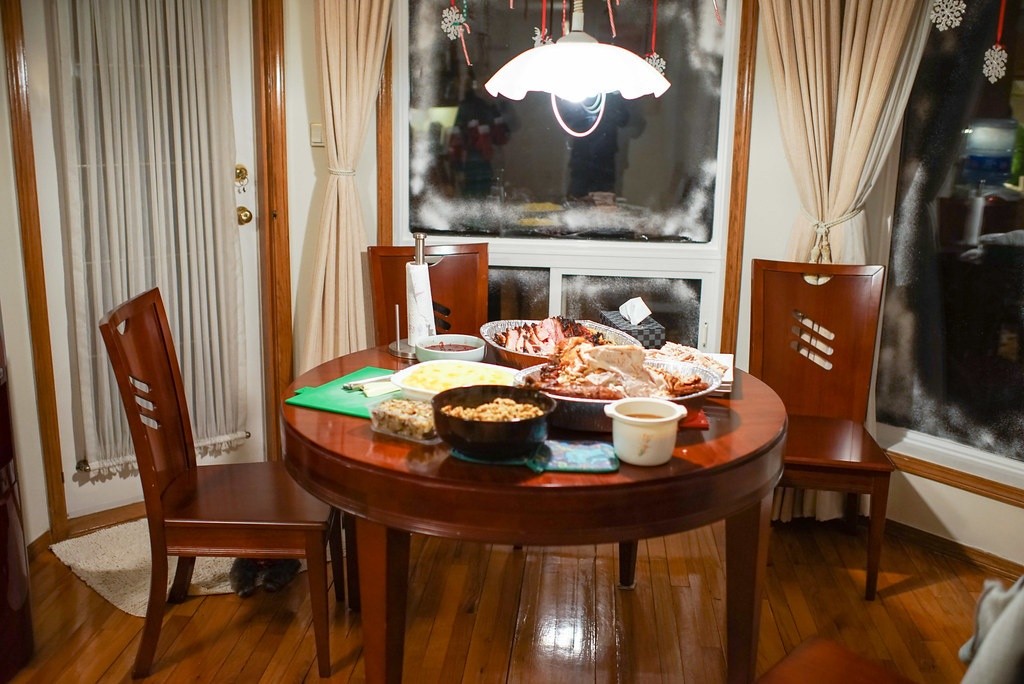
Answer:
281, 339, 788, 684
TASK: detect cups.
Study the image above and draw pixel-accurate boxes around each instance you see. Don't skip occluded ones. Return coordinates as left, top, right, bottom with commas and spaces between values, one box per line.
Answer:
603, 397, 687, 466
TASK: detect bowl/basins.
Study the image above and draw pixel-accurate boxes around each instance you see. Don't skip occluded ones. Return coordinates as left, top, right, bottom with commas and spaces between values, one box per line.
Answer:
431, 383, 557, 459
390, 359, 519, 400
513, 356, 721, 431
479, 319, 643, 369
415, 334, 486, 363
367, 392, 439, 442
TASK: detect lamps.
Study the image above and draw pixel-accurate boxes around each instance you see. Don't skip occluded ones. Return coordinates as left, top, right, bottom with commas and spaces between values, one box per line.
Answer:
484, 0, 671, 139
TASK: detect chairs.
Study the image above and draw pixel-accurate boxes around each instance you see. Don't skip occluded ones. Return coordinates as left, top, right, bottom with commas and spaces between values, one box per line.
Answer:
748, 259, 895, 600
99, 287, 346, 683
366, 242, 489, 347
757, 634, 910, 683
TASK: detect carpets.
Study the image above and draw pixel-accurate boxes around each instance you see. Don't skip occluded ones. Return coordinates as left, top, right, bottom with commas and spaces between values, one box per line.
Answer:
49, 516, 347, 618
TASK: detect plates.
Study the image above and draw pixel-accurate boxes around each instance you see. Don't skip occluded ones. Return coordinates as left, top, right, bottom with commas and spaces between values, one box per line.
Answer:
702, 353, 734, 397
